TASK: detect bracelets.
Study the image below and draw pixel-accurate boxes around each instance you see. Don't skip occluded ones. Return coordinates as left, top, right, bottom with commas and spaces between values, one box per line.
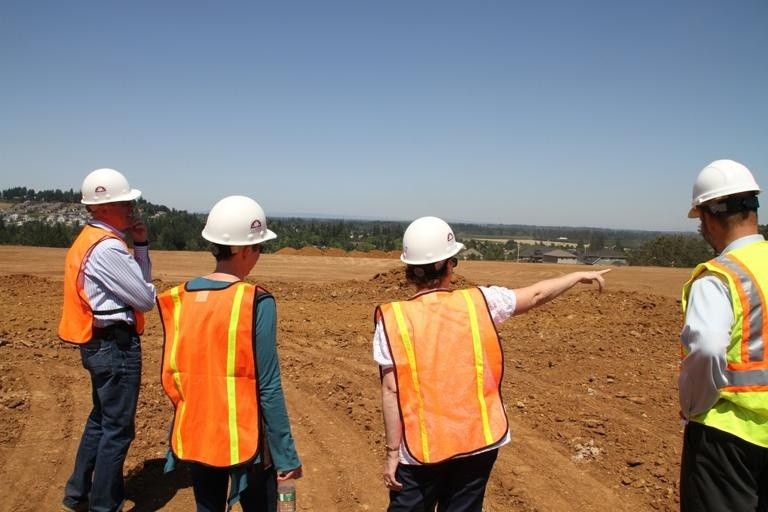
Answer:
384, 444, 400, 451
133, 238, 149, 247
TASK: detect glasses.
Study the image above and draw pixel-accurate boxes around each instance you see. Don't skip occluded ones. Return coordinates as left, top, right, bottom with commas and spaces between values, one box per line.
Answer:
446, 257, 458, 267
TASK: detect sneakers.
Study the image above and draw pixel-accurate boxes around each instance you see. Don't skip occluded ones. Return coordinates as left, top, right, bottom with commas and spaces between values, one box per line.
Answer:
61, 499, 90, 512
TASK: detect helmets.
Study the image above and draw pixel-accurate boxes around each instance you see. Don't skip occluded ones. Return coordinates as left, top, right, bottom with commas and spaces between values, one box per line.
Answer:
201, 195, 278, 246
81, 167, 142, 205
400, 216, 465, 265
688, 159, 761, 218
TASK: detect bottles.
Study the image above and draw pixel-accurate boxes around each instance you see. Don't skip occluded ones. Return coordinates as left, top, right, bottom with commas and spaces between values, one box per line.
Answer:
278, 478, 296, 512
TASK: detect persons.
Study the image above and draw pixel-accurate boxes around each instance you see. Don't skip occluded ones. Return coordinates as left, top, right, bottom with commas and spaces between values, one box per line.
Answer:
59, 168, 156, 511
678, 159, 768, 512
158, 193, 302, 510
373, 215, 611, 511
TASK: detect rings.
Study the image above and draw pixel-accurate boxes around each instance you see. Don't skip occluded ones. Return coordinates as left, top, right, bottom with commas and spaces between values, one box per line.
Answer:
386, 481, 390, 485
